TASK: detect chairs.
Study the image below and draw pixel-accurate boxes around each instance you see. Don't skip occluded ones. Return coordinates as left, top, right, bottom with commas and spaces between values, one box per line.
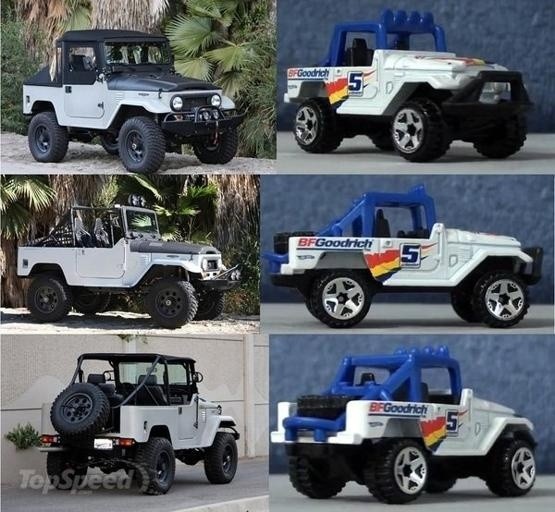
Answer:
374, 208, 392, 238
85, 373, 115, 396
349, 38, 372, 67
421, 382, 429, 402
358, 372, 375, 385
136, 374, 168, 406
71, 216, 93, 248
69, 54, 90, 72
92, 216, 111, 248
